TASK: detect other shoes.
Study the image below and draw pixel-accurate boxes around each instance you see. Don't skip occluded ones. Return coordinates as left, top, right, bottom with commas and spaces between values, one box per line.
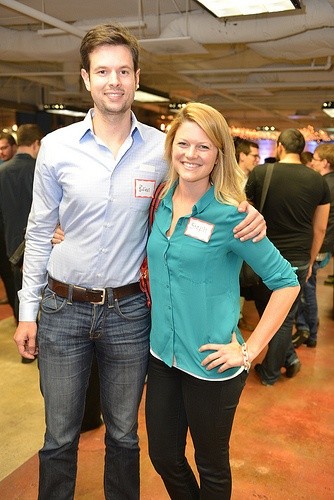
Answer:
254, 363, 262, 377
285, 360, 301, 379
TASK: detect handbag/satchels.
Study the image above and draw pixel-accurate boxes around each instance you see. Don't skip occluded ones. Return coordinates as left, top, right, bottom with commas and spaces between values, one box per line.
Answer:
238, 260, 264, 301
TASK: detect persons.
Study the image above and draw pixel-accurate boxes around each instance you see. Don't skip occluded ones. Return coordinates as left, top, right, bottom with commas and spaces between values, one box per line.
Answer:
52, 102, 301, 500
231, 141, 261, 192
285, 143, 334, 349
0, 131, 18, 319
244, 128, 331, 385
13, 24, 266, 500
1, 124, 43, 364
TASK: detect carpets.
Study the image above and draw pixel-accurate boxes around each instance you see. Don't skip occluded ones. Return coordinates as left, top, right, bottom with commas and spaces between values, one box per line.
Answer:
0, 316, 47, 481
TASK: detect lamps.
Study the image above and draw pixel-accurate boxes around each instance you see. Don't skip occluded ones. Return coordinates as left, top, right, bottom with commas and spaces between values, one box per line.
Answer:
44, 105, 88, 119
321, 101, 334, 119
194, 0, 302, 23
133, 85, 172, 107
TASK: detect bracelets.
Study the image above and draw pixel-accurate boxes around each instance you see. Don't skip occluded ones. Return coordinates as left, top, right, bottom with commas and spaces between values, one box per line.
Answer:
241, 342, 250, 374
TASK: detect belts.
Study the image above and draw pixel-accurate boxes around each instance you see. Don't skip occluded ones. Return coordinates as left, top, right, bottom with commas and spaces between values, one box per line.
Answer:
47, 275, 142, 304
